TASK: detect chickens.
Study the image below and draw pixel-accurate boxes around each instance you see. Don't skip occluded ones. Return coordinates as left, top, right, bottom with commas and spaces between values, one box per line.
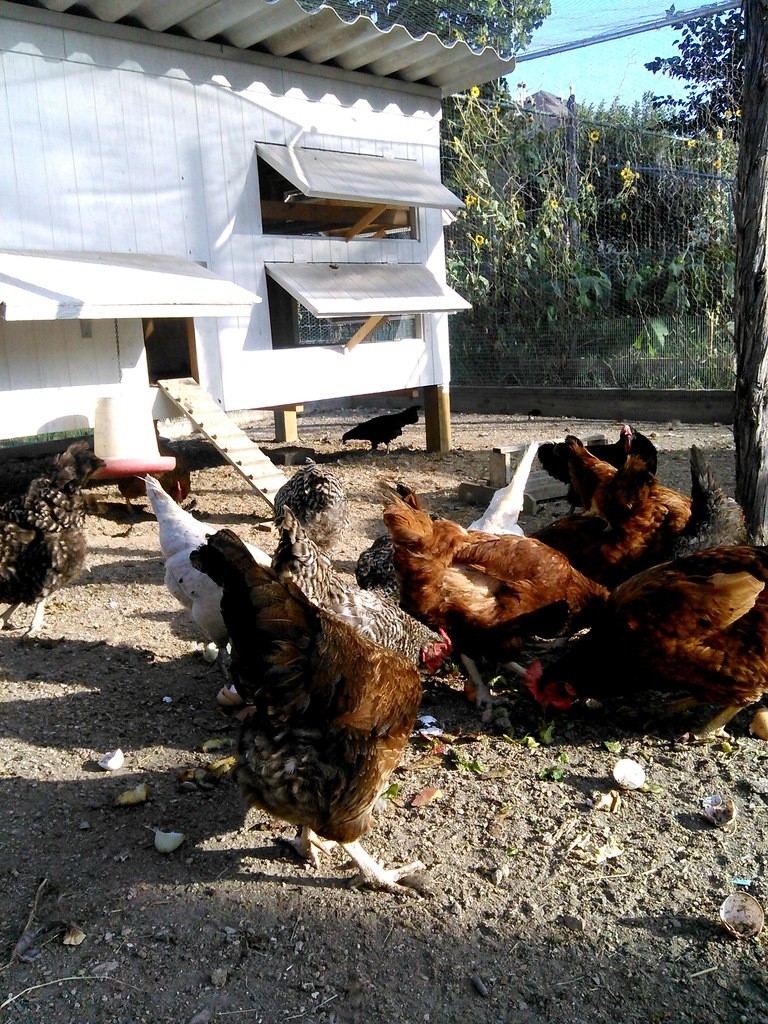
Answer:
0, 403, 768, 899
